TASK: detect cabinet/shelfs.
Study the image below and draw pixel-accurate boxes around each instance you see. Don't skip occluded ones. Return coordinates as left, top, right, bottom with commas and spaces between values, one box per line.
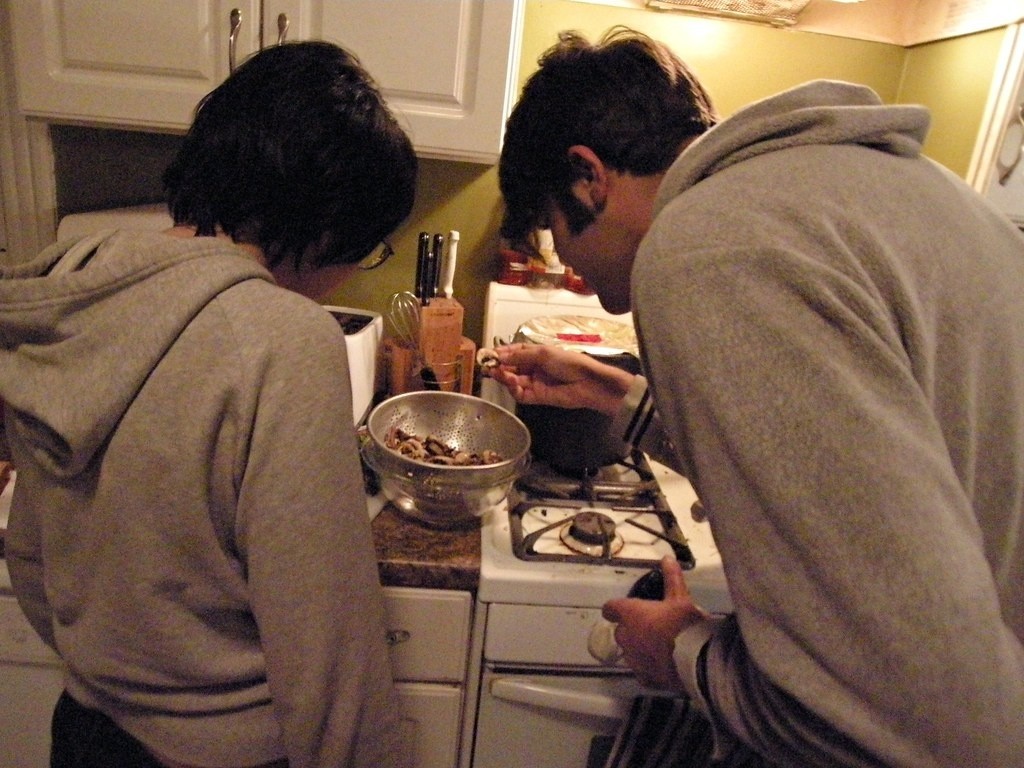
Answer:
2, 0, 526, 169
0, 588, 472, 768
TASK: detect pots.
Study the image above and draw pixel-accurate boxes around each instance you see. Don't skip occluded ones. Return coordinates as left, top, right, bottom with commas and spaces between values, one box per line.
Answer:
512, 315, 640, 469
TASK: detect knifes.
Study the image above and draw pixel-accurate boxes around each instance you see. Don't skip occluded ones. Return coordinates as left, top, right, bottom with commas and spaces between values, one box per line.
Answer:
414, 229, 459, 306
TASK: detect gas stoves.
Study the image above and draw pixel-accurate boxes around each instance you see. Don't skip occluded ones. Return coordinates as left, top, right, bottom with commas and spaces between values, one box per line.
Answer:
478, 450, 736, 616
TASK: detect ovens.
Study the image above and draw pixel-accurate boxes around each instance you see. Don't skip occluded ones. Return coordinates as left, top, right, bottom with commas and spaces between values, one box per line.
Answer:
471, 604, 628, 768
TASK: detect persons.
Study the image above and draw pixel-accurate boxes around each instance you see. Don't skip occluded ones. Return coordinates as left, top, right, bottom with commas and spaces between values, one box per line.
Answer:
481, 27, 1024, 768
0, 40, 417, 768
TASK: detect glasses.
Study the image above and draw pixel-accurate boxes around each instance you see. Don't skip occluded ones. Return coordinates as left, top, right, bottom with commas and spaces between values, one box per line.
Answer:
355, 236, 395, 271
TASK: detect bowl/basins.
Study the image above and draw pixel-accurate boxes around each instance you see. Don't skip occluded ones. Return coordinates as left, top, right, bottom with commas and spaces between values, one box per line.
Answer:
358, 438, 532, 527
367, 389, 532, 484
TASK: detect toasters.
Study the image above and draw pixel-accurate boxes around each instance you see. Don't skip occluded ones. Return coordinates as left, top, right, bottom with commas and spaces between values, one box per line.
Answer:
321, 306, 385, 430
